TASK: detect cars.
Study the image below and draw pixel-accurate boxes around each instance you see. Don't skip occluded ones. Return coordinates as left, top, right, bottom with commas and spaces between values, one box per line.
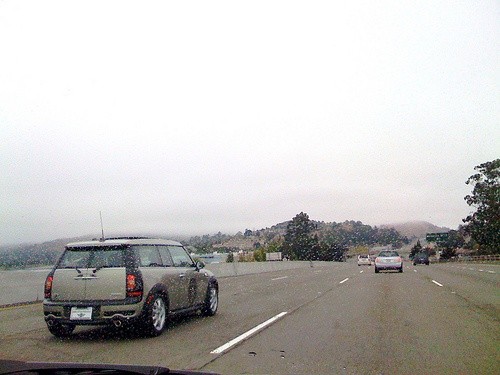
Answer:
357, 254, 377, 266
374, 250, 404, 273
412, 254, 457, 265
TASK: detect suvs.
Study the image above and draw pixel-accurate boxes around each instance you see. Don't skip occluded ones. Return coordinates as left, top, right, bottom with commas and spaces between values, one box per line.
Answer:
42, 238, 220, 336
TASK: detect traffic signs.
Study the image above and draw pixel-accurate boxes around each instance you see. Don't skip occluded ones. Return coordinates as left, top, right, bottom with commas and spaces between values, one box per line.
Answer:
426, 233, 448, 244
